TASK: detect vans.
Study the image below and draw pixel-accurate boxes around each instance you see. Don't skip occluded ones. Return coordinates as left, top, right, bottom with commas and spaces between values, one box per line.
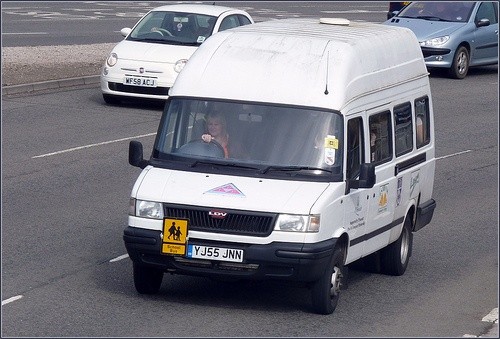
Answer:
123, 17, 437, 315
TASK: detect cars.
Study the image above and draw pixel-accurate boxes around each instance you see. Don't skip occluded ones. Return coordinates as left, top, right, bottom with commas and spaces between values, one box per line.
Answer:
380, 1, 499, 79
100, 3, 255, 105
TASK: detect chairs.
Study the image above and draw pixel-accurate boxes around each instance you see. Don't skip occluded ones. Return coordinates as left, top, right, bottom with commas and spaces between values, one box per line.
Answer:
208, 17, 231, 33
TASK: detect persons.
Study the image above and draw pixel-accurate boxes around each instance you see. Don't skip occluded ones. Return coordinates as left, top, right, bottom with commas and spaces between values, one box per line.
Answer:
200, 110, 234, 159
